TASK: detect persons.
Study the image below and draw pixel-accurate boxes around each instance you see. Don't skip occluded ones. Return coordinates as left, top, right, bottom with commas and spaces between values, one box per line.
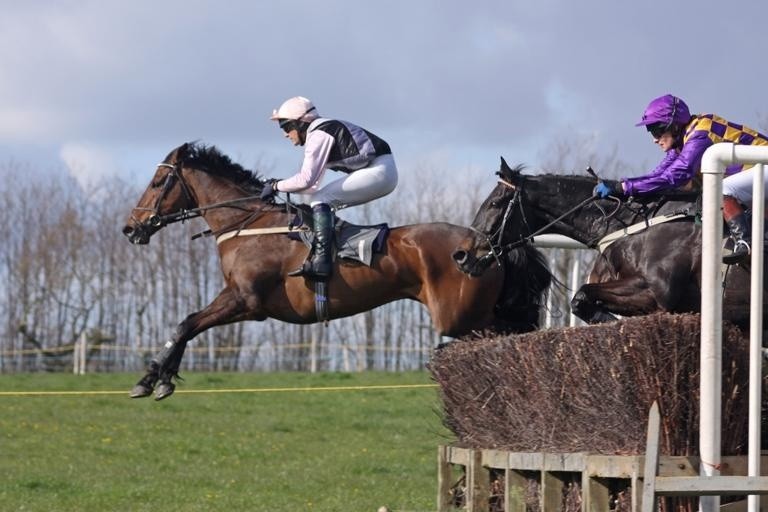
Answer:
589, 93, 768, 268
258, 95, 400, 277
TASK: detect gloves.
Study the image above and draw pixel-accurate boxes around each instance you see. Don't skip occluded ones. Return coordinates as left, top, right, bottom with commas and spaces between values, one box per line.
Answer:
259, 182, 275, 200
592, 182, 625, 198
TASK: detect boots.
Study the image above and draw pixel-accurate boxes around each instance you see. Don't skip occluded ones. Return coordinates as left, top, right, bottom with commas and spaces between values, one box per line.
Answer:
287, 203, 335, 278
722, 196, 754, 263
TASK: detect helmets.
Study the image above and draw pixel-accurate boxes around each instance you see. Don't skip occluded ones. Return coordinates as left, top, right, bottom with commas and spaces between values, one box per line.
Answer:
635, 94, 690, 129
270, 96, 317, 122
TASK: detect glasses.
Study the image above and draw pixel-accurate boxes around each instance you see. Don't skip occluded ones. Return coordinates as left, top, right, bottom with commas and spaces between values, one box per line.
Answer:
651, 124, 670, 138
280, 118, 298, 133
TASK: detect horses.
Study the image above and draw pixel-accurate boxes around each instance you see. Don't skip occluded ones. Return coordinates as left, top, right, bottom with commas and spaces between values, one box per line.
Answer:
121, 137, 574, 402
450, 156, 768, 348
